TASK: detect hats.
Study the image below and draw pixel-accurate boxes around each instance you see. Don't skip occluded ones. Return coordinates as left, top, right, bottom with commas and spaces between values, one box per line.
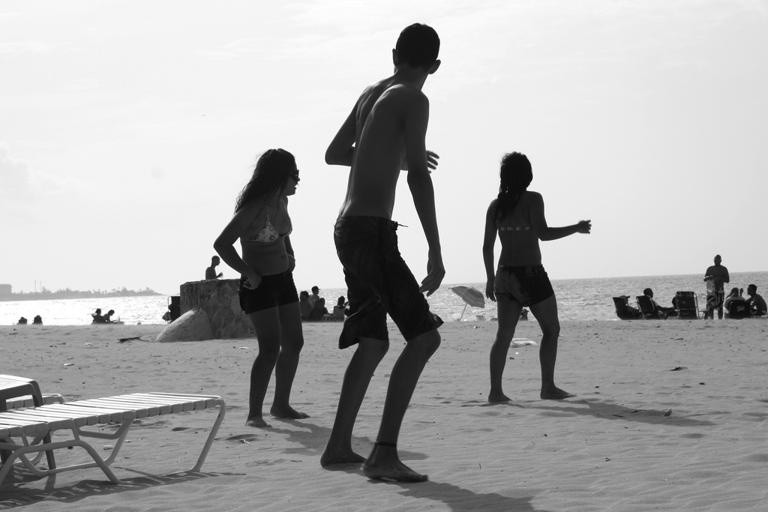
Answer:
312, 286, 320, 291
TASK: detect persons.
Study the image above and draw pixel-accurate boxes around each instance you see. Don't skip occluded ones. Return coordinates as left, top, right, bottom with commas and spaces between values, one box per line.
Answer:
481, 151, 592, 403
298, 285, 349, 321
210, 145, 307, 426
739, 284, 767, 315
98, 309, 114, 322
316, 22, 445, 484
205, 256, 224, 281
644, 289, 677, 320
92, 308, 102, 323
703, 254, 731, 320
725, 287, 745, 311
620, 295, 642, 320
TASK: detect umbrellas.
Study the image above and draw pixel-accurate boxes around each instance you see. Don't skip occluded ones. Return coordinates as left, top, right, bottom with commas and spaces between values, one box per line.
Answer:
449, 285, 485, 320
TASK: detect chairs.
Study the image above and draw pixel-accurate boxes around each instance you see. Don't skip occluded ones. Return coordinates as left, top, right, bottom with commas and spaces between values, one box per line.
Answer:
168, 295, 181, 322
612, 291, 709, 320
0, 374, 226, 494
